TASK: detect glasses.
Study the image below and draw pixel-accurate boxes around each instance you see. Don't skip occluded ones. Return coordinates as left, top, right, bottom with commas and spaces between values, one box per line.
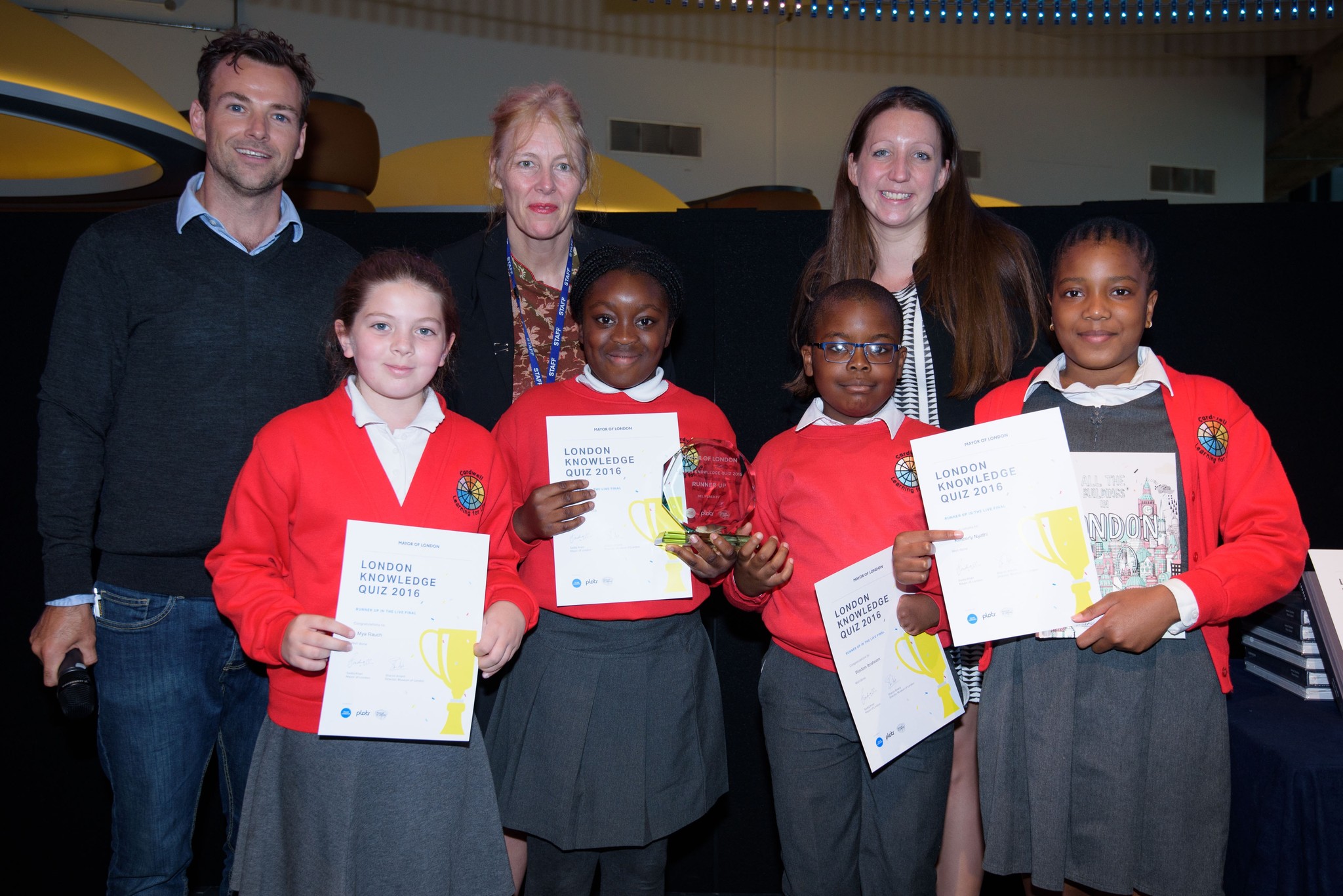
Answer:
809, 342, 902, 364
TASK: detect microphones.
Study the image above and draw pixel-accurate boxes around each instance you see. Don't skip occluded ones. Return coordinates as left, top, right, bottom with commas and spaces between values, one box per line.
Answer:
58, 648, 96, 720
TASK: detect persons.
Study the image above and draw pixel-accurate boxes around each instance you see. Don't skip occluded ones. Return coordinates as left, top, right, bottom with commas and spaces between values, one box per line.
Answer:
207, 245, 536, 896
30, 28, 379, 896
720, 275, 972, 896
778, 83, 1055, 896
425, 77, 742, 896
888, 204, 1312, 896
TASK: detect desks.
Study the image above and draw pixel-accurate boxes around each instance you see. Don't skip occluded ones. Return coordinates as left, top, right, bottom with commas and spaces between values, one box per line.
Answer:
1223, 660, 1343, 896
